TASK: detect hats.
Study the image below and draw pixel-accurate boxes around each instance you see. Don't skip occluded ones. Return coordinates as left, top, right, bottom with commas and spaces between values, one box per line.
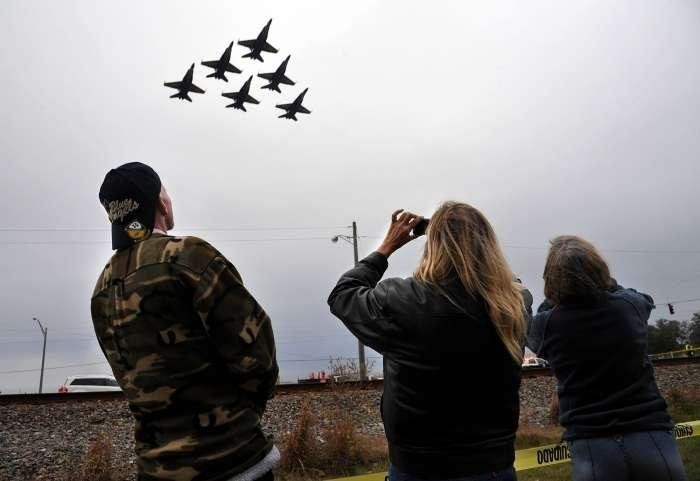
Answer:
99, 163, 161, 251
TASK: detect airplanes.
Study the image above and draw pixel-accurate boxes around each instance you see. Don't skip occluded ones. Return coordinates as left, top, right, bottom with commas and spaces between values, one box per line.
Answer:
165, 17, 310, 121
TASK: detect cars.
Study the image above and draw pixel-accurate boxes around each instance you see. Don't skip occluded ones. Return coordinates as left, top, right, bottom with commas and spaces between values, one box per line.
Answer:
522, 358, 545, 367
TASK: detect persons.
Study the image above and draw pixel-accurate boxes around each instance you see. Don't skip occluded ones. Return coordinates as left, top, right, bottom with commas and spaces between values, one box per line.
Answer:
90, 160, 282, 481
328, 199, 533, 481
524, 234, 689, 481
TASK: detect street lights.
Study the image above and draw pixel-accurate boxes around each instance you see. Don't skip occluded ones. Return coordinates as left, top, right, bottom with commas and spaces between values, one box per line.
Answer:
331, 222, 366, 382
33, 318, 47, 393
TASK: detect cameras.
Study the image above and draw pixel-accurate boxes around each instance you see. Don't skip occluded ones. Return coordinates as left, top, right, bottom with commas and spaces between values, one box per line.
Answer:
414, 216, 431, 238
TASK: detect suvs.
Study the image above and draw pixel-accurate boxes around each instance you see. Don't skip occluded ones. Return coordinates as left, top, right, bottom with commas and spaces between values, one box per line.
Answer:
59, 375, 123, 393
